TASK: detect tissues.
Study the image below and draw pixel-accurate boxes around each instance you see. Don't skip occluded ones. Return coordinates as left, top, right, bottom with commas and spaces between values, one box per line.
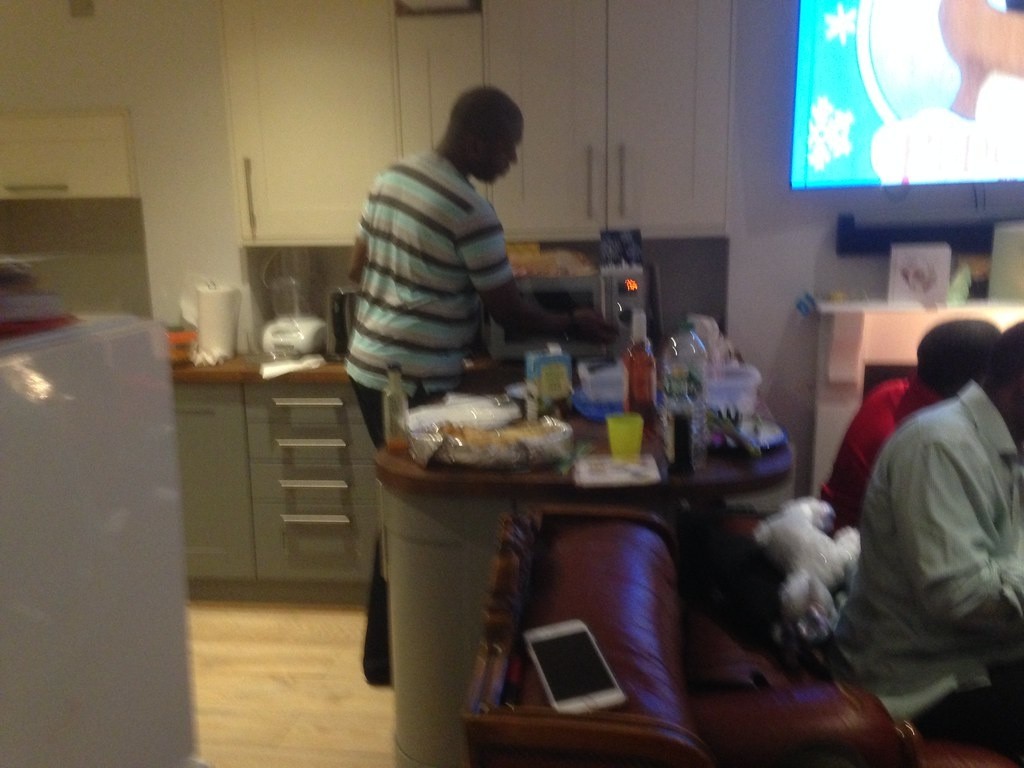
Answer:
524, 341, 573, 402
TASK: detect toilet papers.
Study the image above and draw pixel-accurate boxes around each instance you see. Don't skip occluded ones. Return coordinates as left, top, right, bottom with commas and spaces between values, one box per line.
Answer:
189, 282, 243, 364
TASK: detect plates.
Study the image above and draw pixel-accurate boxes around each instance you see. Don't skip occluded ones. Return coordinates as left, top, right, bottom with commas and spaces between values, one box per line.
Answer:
719, 417, 791, 454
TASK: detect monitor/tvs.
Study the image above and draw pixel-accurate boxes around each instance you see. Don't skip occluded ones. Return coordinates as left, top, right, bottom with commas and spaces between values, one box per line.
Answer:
788, 0, 1024, 190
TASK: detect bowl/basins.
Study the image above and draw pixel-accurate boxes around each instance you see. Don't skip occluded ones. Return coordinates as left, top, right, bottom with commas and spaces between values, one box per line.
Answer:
575, 356, 628, 406
701, 361, 762, 418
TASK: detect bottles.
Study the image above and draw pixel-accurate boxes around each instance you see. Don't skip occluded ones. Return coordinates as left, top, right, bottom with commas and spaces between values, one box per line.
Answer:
656, 319, 710, 464
622, 335, 654, 422
661, 363, 696, 477
380, 363, 410, 453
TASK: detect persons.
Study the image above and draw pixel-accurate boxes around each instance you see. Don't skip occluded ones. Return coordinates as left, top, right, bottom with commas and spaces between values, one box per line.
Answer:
816, 320, 1000, 534
343, 85, 623, 687
835, 322, 1023, 759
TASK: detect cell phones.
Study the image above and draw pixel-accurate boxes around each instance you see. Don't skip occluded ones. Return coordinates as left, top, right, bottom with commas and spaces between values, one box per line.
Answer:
523, 617, 626, 713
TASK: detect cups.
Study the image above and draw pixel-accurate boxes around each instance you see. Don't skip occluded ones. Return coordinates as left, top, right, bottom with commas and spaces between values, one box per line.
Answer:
604, 410, 644, 458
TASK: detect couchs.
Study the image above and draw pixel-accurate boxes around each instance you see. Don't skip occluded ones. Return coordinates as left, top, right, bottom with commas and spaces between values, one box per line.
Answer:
451, 499, 1018, 767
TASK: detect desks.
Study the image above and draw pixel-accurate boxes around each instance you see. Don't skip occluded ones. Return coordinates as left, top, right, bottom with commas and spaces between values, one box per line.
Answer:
374, 336, 800, 768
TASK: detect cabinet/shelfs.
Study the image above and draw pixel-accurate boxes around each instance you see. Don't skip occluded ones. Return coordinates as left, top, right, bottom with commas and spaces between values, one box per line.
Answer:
174, 369, 255, 608
237, 371, 384, 609
